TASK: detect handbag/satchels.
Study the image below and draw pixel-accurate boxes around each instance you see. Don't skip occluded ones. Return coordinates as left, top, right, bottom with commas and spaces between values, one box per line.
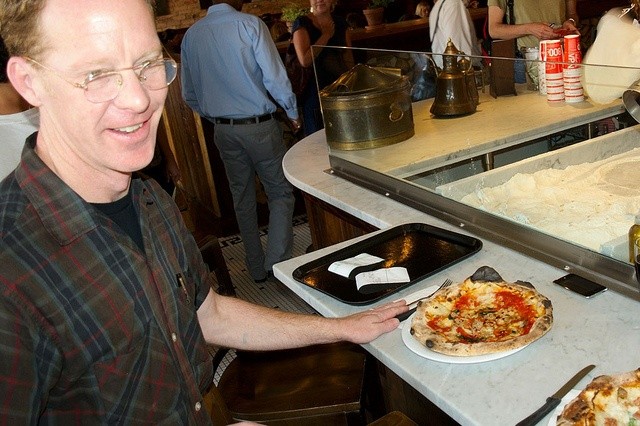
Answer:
409, 54, 441, 101
480, 14, 522, 67
285, 41, 311, 96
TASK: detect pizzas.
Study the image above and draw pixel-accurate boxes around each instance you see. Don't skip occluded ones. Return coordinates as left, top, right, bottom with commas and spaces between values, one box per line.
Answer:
410, 266, 555, 358
555, 366, 640, 426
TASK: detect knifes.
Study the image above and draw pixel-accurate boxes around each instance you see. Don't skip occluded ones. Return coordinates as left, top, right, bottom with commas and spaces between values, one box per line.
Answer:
517, 364, 596, 426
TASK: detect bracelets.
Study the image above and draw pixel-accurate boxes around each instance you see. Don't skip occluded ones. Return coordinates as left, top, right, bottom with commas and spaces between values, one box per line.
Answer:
569, 18, 577, 26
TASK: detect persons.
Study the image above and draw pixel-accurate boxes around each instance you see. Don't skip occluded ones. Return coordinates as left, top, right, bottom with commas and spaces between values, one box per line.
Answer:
0, 0, 410, 426
179, 1, 302, 283
487, 0, 581, 59
428, 0, 482, 70
286, 1, 355, 135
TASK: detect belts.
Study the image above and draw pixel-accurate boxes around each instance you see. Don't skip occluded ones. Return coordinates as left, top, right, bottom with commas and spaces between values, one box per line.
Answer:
215, 111, 279, 125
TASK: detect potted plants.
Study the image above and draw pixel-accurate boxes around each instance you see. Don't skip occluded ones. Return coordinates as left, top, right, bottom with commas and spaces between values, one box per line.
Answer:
282, 3, 310, 35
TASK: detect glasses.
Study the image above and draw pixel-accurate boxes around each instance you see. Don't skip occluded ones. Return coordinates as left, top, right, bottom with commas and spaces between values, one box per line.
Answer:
20, 43, 179, 104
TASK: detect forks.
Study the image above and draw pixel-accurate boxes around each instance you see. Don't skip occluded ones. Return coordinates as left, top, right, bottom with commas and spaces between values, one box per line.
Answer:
393, 278, 453, 323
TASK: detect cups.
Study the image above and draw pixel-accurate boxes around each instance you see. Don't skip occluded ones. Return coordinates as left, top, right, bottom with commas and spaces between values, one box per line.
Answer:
538, 41, 546, 95
562, 34, 585, 104
545, 39, 564, 102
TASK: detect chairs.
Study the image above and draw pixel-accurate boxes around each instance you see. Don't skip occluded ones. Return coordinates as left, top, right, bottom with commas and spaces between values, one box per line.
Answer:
198, 233, 369, 426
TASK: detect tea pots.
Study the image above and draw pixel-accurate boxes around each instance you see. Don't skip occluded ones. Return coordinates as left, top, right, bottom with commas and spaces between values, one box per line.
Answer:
457, 51, 479, 106
421, 38, 477, 117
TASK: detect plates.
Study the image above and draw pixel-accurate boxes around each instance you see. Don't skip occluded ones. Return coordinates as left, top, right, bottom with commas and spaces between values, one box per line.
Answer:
402, 310, 527, 366
547, 389, 584, 426
